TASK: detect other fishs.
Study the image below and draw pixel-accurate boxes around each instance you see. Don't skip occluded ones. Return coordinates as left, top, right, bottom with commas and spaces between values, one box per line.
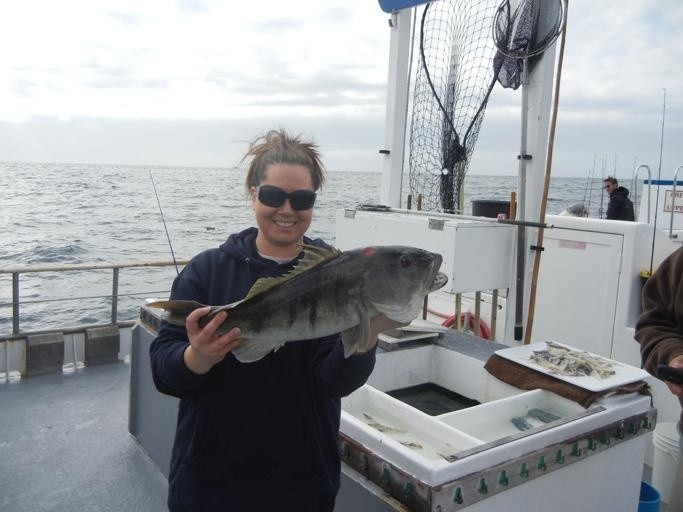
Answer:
146, 243, 449, 365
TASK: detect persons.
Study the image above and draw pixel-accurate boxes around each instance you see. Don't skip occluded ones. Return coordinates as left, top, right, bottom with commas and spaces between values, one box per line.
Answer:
602, 177, 634, 221
145, 124, 382, 510
632, 244, 683, 406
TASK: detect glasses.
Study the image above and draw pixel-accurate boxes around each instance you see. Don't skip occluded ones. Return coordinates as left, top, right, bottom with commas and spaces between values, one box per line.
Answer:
254, 184, 317, 211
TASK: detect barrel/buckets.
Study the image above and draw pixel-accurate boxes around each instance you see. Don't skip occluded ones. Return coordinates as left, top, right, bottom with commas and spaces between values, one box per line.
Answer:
652, 421, 683, 512
638, 480, 661, 512
